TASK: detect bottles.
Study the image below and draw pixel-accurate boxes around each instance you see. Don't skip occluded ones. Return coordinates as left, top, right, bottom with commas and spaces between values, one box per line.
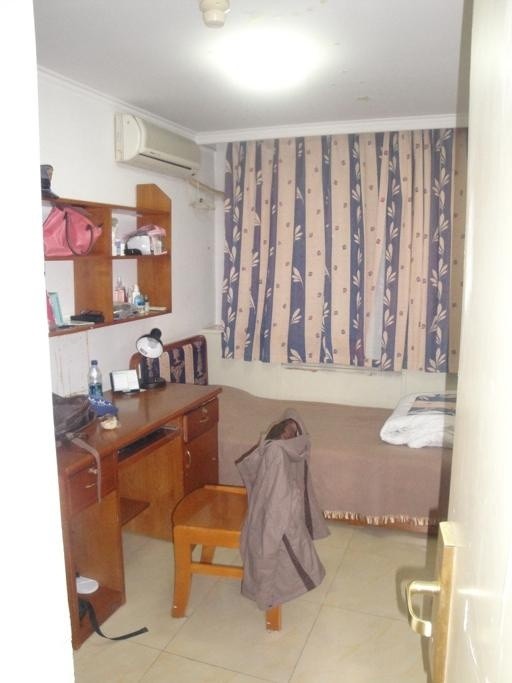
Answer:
86, 359, 105, 405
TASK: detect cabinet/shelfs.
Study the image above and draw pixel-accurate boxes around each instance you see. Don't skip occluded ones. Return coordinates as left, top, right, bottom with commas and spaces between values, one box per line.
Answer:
41, 185, 172, 339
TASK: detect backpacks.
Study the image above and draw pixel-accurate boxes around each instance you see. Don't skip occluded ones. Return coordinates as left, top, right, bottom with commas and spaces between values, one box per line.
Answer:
51, 393, 97, 440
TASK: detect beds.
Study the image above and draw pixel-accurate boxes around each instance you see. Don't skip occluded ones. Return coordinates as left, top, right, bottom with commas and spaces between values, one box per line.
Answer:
129, 334, 453, 535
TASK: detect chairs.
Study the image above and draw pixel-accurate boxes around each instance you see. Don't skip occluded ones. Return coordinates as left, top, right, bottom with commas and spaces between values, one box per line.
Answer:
170, 407, 300, 631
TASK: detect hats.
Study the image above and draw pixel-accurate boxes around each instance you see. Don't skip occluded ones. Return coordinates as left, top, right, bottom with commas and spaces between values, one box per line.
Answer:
40, 165, 59, 200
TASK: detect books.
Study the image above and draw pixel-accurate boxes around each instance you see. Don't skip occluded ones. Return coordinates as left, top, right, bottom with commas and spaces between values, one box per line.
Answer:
47, 292, 63, 332
62, 319, 95, 327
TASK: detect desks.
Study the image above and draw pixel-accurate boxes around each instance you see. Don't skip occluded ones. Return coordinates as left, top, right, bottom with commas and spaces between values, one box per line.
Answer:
56, 380, 223, 650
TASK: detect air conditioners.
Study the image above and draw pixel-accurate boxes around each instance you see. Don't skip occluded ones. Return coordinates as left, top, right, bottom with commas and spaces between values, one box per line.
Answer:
114, 112, 203, 181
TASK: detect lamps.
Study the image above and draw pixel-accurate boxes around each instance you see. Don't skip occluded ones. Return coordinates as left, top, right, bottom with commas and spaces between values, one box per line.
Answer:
136, 328, 167, 390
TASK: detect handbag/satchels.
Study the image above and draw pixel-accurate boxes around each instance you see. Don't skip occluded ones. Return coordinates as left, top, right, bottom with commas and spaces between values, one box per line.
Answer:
43, 205, 105, 257
